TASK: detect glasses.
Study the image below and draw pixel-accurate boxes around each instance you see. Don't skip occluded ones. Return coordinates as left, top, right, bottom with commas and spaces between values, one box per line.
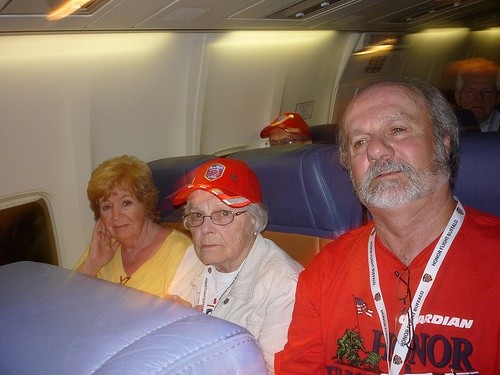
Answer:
181, 210, 246, 227
394, 269, 416, 349
264, 140, 296, 148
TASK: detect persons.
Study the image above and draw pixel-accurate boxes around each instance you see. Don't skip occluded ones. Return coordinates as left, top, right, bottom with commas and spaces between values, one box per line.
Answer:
163, 158, 305, 375
455, 58, 500, 133
259, 112, 313, 148
274, 77, 500, 375
71, 155, 192, 300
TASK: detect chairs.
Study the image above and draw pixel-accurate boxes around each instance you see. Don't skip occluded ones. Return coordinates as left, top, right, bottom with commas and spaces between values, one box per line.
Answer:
0, 261, 269, 375
145, 109, 500, 269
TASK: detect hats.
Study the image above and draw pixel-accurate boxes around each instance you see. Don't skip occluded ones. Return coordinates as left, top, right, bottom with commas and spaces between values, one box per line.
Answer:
171, 157, 262, 208
260, 113, 312, 139
